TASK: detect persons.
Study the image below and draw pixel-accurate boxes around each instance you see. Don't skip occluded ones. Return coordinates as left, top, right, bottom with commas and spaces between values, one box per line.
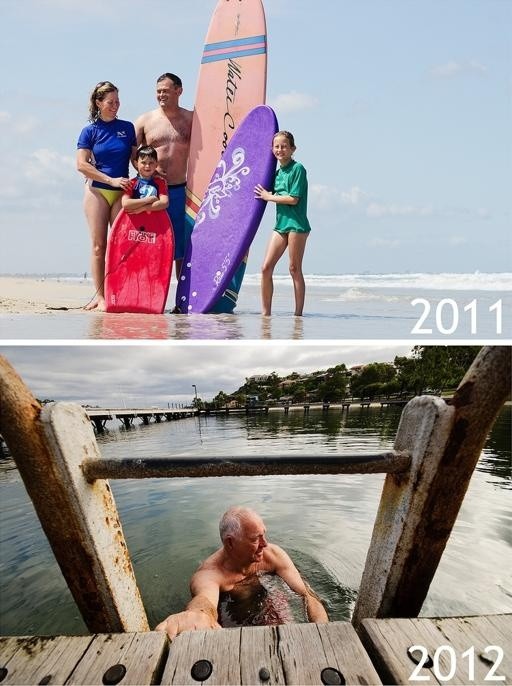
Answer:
75, 81, 138, 310
253, 131, 311, 316
121, 146, 170, 215
133, 72, 193, 281
153, 505, 329, 643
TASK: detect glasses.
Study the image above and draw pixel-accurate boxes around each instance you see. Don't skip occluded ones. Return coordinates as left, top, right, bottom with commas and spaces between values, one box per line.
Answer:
94, 81, 110, 92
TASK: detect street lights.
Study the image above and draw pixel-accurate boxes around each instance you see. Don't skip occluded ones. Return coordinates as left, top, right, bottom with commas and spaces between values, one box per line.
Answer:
191, 385, 197, 408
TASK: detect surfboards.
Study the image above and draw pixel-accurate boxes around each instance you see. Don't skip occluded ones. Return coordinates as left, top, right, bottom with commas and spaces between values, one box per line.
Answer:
104, 202, 175, 315
174, 0, 278, 313
